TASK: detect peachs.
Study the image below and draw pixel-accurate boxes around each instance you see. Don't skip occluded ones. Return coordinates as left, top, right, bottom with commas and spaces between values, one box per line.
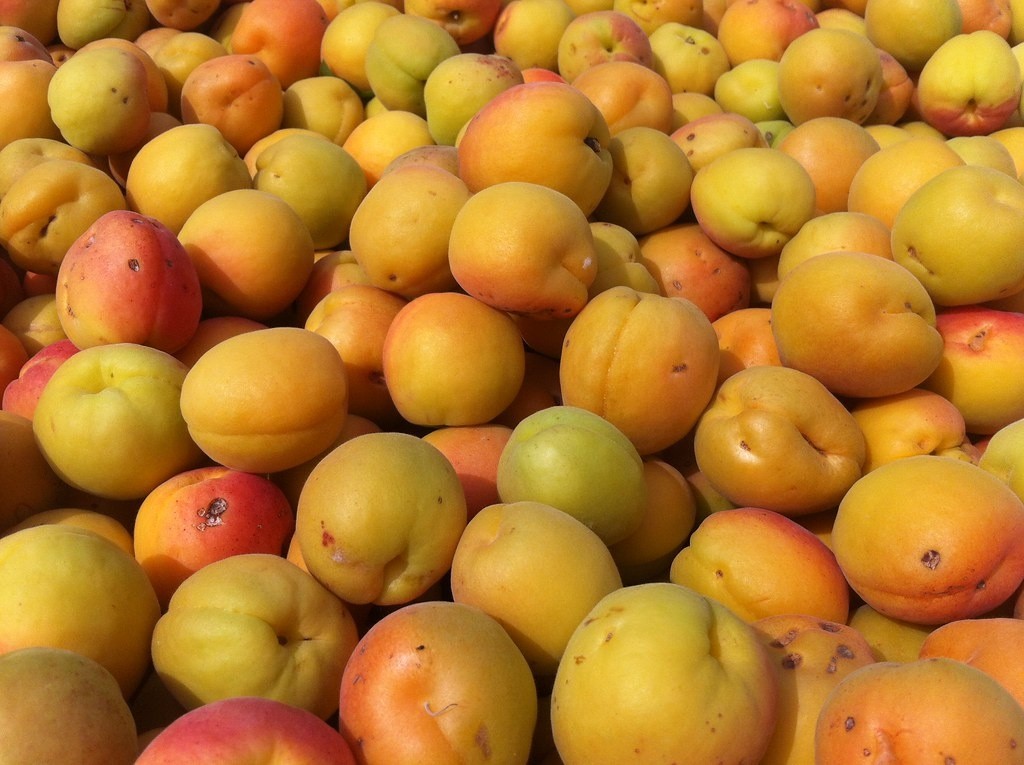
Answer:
0, 0, 1024, 765
